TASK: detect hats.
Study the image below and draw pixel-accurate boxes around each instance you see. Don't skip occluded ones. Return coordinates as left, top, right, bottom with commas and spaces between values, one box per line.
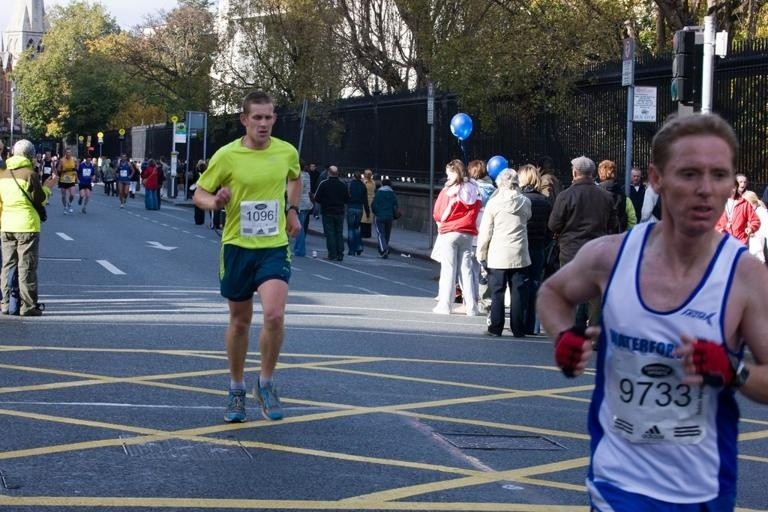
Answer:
12, 138, 36, 159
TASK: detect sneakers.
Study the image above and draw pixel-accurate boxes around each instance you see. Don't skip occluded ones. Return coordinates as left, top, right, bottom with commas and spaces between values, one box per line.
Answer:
292, 246, 393, 262
119, 201, 129, 209
314, 215, 320, 220
251, 375, 285, 422
1, 306, 43, 317
45, 202, 50, 207
78, 198, 87, 214
63, 202, 74, 216
222, 385, 249, 423
431, 288, 602, 351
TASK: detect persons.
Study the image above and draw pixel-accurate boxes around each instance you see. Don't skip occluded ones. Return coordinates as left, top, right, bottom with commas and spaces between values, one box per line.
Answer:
191, 92, 301, 422
536, 112, 765, 511
1, 138, 57, 317
430, 155, 661, 340
292, 162, 401, 262
715, 174, 766, 264
31, 149, 207, 225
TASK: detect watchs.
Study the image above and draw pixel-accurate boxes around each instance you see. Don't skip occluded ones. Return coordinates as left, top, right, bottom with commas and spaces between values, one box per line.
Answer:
734, 361, 751, 390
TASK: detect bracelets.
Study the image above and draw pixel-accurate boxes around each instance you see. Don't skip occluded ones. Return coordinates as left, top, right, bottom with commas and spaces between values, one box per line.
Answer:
286, 205, 300, 214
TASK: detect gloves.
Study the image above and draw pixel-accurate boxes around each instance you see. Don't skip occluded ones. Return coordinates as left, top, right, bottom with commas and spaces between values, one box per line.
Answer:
667, 331, 744, 393
552, 324, 602, 381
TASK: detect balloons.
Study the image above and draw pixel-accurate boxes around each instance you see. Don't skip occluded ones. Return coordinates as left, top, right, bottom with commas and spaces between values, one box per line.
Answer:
447, 112, 473, 142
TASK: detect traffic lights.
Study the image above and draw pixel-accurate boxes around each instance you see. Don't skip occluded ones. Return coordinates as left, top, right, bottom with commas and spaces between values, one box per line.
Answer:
669, 26, 696, 106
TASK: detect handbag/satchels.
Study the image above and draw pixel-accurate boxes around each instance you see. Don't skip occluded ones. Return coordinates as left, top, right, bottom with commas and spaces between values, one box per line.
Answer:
392, 204, 402, 220
6, 264, 22, 317
28, 197, 48, 223
428, 231, 443, 264
539, 237, 562, 279
142, 177, 150, 186
